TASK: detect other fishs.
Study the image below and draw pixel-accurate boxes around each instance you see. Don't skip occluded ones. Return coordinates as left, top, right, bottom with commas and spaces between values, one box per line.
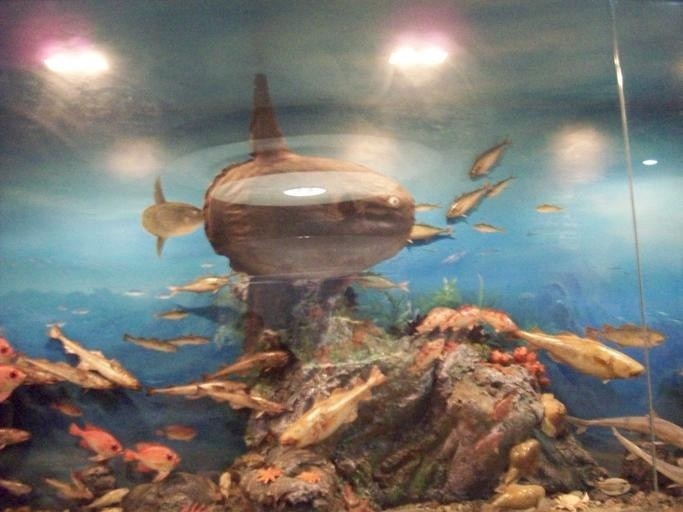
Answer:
0, 305, 683, 512
